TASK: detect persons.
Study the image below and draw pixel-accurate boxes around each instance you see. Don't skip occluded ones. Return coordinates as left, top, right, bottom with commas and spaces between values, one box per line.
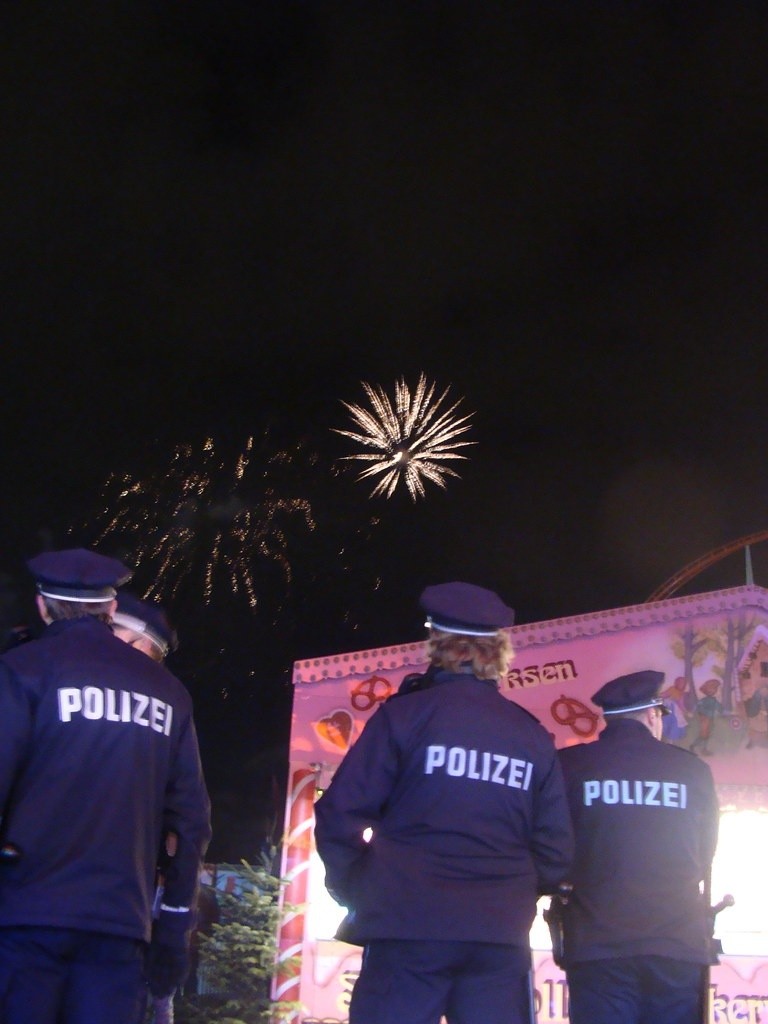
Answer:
309, 578, 576, 1024
0, 548, 215, 1024
549, 667, 726, 1024
107, 589, 192, 1023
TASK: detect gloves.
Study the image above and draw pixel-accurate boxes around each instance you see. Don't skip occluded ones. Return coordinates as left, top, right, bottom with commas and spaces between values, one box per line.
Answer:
145, 909, 191, 998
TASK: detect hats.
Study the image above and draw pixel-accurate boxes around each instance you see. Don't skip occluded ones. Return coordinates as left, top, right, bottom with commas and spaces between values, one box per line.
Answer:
109, 592, 178, 656
422, 582, 513, 635
592, 670, 673, 716
28, 549, 133, 603
